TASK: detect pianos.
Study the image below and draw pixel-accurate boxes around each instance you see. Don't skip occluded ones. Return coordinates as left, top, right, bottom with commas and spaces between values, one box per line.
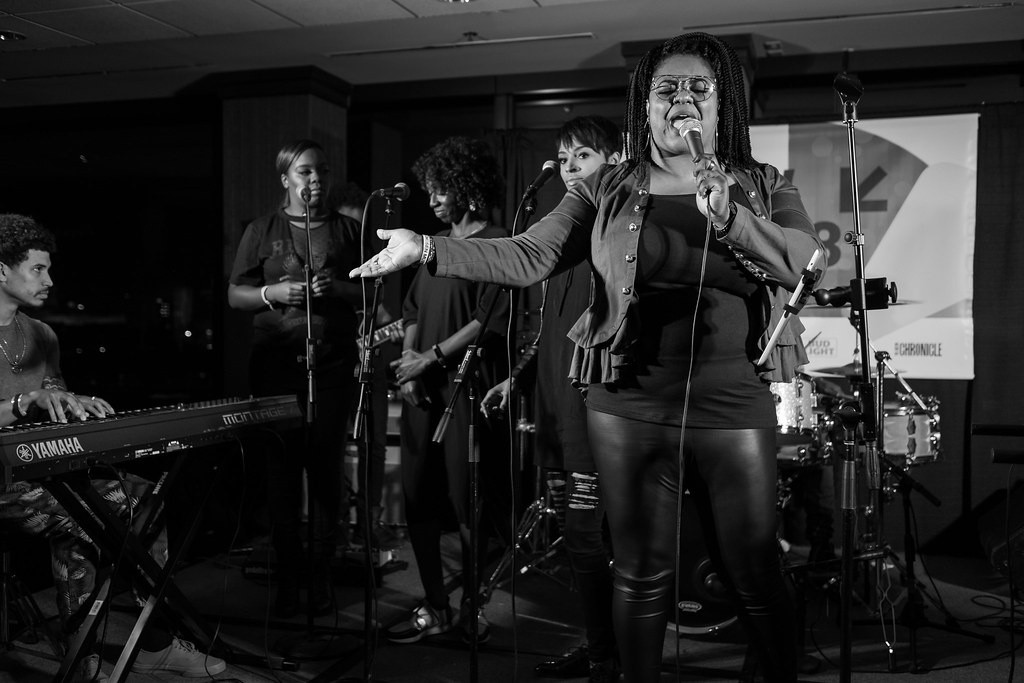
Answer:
0, 393, 301, 683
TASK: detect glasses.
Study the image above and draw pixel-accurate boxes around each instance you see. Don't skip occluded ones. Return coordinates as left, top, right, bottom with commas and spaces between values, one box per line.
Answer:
648, 75, 718, 104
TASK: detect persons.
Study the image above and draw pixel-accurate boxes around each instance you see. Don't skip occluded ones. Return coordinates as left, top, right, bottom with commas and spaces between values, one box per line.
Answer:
227, 139, 375, 617
0, 213, 226, 683
348, 32, 828, 683
382, 133, 520, 642
480, 116, 624, 683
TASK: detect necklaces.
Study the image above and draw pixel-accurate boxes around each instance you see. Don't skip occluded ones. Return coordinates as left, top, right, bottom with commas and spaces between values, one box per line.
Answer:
0, 315, 26, 374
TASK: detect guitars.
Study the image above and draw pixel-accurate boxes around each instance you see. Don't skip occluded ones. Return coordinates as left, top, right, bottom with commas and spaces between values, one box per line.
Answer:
356, 319, 404, 351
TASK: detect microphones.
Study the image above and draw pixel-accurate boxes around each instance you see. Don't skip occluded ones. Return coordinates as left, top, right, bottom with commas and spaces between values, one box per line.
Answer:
523, 160, 560, 200
372, 183, 410, 200
704, 571, 729, 598
296, 185, 311, 202
679, 118, 704, 165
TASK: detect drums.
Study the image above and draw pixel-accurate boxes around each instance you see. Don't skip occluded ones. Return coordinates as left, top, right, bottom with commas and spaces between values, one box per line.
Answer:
600, 472, 793, 635
769, 374, 819, 460
879, 401, 941, 467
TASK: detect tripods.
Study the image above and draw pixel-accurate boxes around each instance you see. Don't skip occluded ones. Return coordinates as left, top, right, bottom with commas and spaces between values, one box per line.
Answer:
806, 99, 997, 674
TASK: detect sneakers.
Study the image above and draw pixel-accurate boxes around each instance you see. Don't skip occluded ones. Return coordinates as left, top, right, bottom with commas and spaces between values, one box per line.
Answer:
78, 653, 109, 683
131, 635, 227, 678
386, 602, 453, 642
460, 597, 490, 644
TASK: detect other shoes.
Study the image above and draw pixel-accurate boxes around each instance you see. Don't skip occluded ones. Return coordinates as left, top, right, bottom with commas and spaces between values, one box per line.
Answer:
534, 639, 592, 677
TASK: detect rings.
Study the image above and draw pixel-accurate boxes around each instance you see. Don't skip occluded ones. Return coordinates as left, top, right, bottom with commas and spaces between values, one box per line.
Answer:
708, 160, 715, 170
91, 396, 96, 400
375, 260, 378, 264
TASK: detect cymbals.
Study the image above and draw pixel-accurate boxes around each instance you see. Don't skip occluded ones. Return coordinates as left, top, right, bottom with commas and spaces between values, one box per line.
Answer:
814, 362, 907, 378
798, 369, 845, 378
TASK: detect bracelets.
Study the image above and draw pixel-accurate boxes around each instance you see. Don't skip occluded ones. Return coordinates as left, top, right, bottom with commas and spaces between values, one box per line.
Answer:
12, 393, 27, 418
419, 234, 435, 265
261, 285, 277, 312
431, 344, 444, 364
